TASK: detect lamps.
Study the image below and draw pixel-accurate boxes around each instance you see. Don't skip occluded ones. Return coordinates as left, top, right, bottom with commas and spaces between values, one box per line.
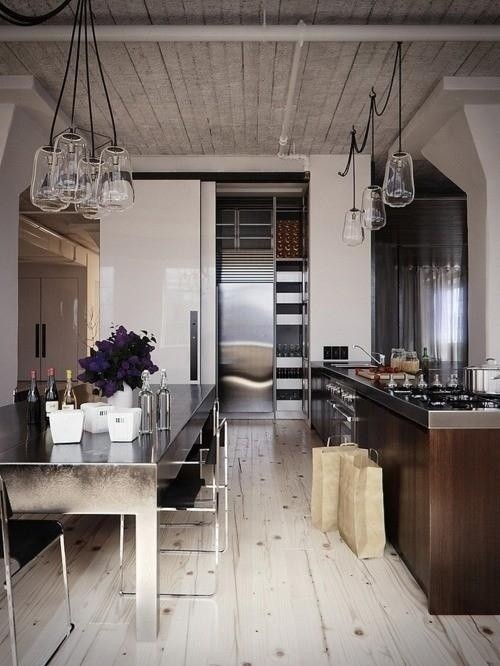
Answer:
343, 126, 363, 246
380, 41, 416, 209
359, 95, 387, 231
29, 1, 136, 220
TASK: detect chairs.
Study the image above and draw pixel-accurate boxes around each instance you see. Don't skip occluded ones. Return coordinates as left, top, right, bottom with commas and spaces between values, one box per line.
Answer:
0, 476, 75, 666
119, 401, 228, 597
13, 387, 33, 403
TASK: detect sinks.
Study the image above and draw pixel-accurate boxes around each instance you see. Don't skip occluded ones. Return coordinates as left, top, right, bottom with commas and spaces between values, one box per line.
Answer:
331, 363, 377, 367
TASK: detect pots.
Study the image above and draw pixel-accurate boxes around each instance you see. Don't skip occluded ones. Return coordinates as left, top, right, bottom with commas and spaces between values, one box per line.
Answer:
463, 358, 500, 395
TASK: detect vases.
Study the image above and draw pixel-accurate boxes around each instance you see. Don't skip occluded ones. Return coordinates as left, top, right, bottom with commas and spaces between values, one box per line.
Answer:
106, 382, 134, 412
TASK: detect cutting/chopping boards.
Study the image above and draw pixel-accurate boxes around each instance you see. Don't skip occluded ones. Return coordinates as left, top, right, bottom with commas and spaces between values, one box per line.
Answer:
357, 367, 416, 380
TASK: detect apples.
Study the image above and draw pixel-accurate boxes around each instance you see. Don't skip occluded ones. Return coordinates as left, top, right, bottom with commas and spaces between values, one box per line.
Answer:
356, 369, 363, 375
374, 375, 380, 381
377, 366, 399, 373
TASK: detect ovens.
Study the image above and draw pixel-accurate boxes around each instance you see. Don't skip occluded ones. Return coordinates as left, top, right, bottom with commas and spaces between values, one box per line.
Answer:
326, 380, 356, 446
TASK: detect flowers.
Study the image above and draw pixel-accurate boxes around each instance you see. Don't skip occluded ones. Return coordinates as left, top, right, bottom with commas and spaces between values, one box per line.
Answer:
75, 311, 159, 398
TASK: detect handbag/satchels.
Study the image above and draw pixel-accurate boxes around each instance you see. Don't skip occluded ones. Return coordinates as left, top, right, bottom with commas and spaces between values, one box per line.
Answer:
311, 434, 386, 559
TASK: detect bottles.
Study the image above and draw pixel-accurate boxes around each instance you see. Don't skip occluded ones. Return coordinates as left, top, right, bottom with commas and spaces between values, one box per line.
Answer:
45, 368, 59, 422
138, 369, 153, 435
422, 347, 430, 373
27, 370, 41, 424
156, 369, 171, 430
276, 343, 301, 357
391, 348, 419, 374
62, 370, 78, 410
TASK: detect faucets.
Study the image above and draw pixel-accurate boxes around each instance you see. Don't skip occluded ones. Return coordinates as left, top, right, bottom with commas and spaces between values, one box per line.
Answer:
352, 344, 385, 367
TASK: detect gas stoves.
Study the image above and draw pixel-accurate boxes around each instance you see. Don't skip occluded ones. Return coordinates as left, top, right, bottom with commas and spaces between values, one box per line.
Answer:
385, 383, 500, 411
